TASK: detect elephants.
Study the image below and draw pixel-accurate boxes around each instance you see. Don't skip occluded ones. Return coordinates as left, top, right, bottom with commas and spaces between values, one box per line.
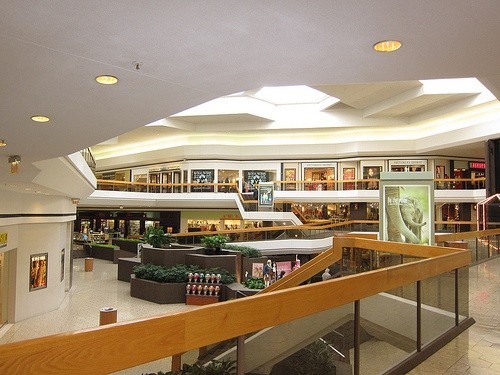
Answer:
385, 186, 427, 244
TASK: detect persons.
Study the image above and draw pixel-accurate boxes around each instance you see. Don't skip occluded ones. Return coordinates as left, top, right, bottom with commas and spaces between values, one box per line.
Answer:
263, 260, 272, 289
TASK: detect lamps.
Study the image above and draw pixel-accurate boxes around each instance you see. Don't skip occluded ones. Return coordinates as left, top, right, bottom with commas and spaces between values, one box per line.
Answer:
0, 139, 7, 147
8, 154, 22, 173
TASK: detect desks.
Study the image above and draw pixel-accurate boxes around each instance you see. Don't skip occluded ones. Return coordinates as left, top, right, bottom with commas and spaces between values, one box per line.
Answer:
226, 283, 263, 299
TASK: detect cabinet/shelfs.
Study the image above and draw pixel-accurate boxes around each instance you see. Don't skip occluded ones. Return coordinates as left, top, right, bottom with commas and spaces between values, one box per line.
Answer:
186, 281, 222, 306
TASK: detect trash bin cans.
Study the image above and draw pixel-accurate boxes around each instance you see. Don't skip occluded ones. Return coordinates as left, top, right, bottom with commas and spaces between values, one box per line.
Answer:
100, 308, 117, 327
85, 257, 94, 272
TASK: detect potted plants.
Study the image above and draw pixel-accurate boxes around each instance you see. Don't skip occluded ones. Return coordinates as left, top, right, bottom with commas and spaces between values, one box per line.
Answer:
158, 234, 175, 248
200, 235, 228, 255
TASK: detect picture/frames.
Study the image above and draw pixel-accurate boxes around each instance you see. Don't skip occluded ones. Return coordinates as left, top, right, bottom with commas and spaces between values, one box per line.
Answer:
28, 252, 48, 291
